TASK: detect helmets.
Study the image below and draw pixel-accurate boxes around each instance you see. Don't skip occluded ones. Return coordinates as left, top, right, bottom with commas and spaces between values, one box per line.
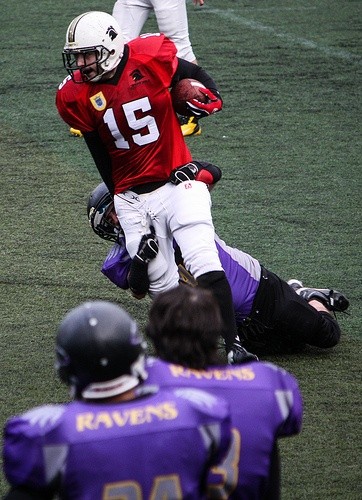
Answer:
85, 180, 125, 246
53, 301, 151, 400
62, 9, 126, 85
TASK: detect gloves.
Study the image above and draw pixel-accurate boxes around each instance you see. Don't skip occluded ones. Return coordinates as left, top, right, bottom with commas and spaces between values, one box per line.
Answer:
183, 87, 224, 120
138, 232, 159, 271
167, 161, 201, 186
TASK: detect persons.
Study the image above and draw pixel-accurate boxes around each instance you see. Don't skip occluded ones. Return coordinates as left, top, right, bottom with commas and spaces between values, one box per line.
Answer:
0, 285, 304, 500
87, 161, 349, 357
56, 10, 258, 365
70, 0, 205, 138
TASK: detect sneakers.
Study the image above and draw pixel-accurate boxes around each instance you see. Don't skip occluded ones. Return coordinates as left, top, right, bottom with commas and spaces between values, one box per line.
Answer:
67, 127, 84, 138
179, 117, 202, 138
293, 286, 351, 313
287, 280, 303, 293
225, 340, 260, 364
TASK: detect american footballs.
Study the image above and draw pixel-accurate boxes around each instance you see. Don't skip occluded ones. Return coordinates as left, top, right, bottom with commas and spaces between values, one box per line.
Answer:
170, 78, 208, 116
